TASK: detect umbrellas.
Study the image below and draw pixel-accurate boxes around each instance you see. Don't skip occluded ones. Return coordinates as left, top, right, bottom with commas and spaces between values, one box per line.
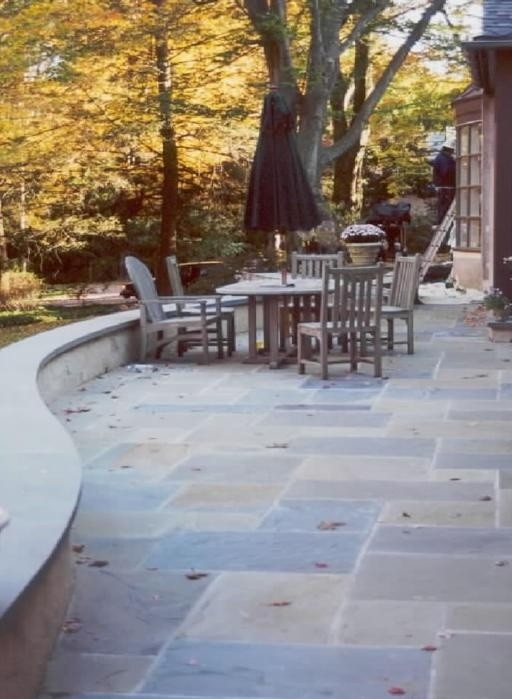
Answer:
242, 84, 322, 358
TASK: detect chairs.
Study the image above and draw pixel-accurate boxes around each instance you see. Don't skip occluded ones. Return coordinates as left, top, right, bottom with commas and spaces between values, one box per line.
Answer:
124, 250, 423, 383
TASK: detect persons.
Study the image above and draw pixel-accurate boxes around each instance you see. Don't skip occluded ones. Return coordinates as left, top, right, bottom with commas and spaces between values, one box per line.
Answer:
432, 144, 456, 226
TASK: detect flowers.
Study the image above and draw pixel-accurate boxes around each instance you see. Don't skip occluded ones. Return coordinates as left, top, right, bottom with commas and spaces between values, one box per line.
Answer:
477, 287, 511, 324
339, 223, 388, 245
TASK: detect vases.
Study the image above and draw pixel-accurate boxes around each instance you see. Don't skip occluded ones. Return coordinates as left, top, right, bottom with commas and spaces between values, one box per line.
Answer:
345, 243, 384, 268
487, 322, 511, 345
